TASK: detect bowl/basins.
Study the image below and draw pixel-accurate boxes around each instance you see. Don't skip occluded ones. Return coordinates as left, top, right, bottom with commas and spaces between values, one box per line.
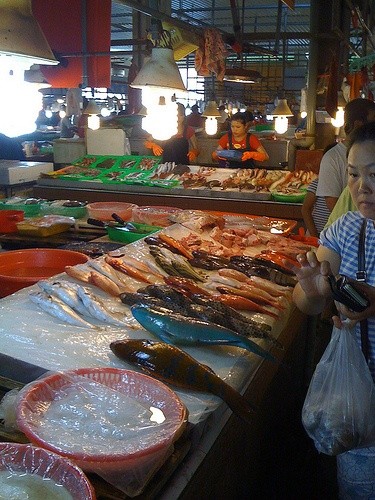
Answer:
46, 201, 87, 219
0, 196, 47, 217
86, 201, 138, 221
133, 205, 183, 227
290, 137, 315, 151
0, 210, 24, 233
0, 248, 91, 299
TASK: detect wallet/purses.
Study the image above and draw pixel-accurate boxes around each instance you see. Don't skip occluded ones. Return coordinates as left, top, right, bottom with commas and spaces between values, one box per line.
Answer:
326, 274, 370, 313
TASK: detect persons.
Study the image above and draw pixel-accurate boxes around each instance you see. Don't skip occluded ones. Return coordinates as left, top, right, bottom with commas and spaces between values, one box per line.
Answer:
144, 102, 200, 165
35, 109, 62, 130
186, 104, 202, 128
59, 100, 91, 137
289, 97, 375, 500
218, 105, 228, 129
211, 111, 269, 169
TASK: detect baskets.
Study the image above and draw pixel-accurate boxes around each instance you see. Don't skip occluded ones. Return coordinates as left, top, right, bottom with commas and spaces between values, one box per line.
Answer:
17, 366, 188, 472
56, 154, 179, 189
0, 442, 95, 500
0, 197, 183, 244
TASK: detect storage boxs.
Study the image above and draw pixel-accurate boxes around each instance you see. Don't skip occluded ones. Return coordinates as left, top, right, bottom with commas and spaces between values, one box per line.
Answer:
108, 223, 162, 242
147, 20, 201, 60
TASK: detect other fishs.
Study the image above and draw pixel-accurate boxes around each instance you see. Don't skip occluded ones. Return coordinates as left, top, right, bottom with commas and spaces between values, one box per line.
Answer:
109, 338, 257, 424
1, 195, 311, 364
65, 157, 313, 194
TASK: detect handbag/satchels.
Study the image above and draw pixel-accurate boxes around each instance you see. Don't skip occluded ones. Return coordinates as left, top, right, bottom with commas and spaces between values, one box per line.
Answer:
302, 321, 375, 457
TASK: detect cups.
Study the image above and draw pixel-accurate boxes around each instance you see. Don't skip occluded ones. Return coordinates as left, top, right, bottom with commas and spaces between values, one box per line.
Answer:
24, 143, 32, 156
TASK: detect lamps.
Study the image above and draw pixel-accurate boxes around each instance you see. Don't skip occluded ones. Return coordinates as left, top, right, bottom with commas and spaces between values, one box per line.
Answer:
201, 0, 221, 136
0, 0, 61, 138
224, 67, 259, 83
129, 17, 187, 141
270, 3, 293, 134
83, 99, 101, 130
331, 89, 348, 128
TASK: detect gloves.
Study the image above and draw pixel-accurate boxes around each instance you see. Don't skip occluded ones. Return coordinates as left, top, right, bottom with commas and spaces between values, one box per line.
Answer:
212, 152, 227, 161
145, 141, 164, 157
187, 148, 198, 161
241, 152, 265, 162
